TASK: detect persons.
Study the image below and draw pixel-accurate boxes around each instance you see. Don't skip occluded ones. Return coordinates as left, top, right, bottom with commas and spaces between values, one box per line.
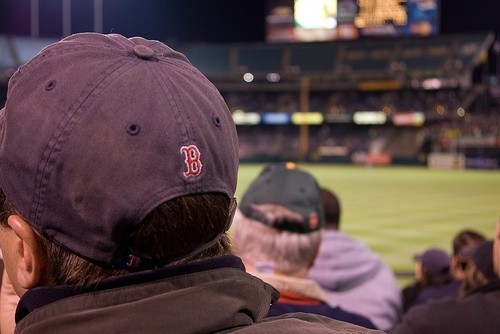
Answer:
389, 218, 500, 333
227, 160, 383, 330
0, 32, 390, 334
309, 186, 403, 332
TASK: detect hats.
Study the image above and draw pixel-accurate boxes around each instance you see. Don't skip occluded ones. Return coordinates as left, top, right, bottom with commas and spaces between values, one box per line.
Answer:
414, 250, 450, 276
241, 162, 325, 233
0, 32, 238, 267
465, 242, 496, 280
